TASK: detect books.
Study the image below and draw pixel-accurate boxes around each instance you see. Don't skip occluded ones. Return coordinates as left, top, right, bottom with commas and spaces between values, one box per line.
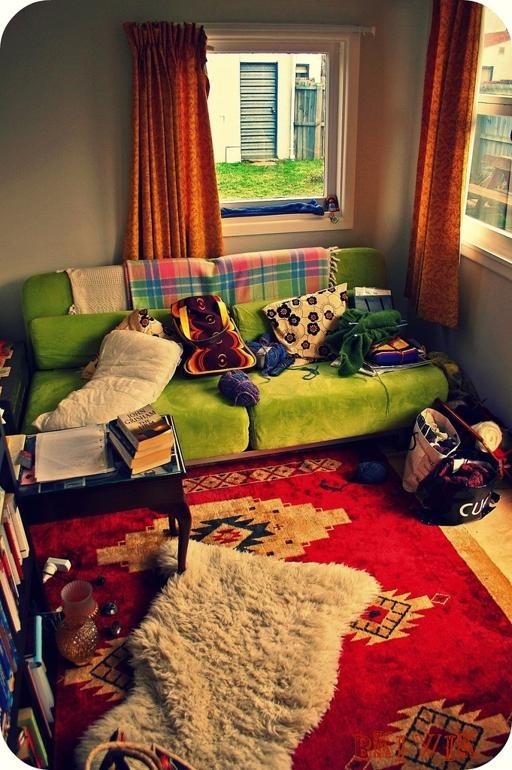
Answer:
5, 434, 26, 480
106, 425, 171, 469
0, 487, 30, 747
103, 423, 117, 472
108, 419, 173, 459
105, 441, 172, 475
35, 425, 107, 483
14, 660, 58, 768
117, 405, 173, 450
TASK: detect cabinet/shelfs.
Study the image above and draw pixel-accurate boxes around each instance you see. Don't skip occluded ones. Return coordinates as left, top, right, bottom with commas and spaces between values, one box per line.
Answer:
0, 418, 57, 770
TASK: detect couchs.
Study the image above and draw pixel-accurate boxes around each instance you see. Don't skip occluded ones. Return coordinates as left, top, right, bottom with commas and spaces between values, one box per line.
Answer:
21, 247, 449, 467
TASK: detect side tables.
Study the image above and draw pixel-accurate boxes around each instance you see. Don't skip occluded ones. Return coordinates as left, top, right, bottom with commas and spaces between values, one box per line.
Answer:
18, 414, 192, 573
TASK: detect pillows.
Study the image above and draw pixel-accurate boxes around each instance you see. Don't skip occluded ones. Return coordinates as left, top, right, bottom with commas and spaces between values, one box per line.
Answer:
170, 295, 257, 376
28, 309, 172, 370
260, 283, 348, 367
231, 296, 295, 344
31, 329, 184, 432
367, 333, 419, 366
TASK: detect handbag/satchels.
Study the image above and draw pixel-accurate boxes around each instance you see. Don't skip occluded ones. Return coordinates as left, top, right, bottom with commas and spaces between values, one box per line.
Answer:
416, 457, 500, 526
402, 407, 461, 494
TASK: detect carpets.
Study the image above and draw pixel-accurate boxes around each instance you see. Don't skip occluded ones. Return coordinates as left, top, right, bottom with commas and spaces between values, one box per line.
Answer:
28, 440, 512, 770
